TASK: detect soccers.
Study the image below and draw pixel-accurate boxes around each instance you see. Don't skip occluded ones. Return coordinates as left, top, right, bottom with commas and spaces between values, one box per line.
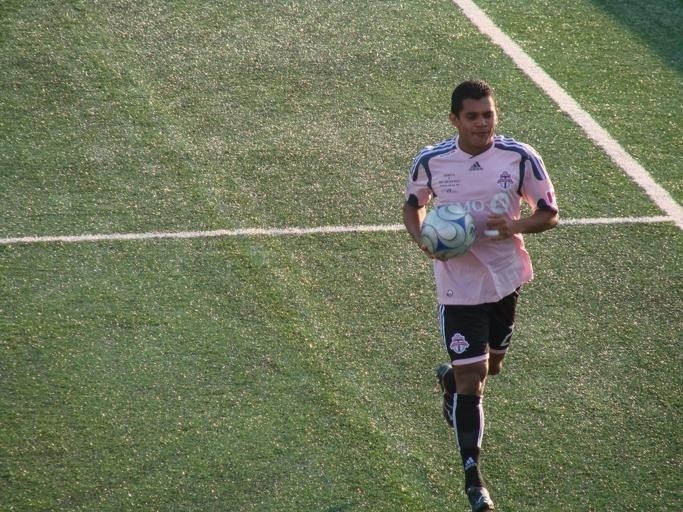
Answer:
421, 205, 475, 261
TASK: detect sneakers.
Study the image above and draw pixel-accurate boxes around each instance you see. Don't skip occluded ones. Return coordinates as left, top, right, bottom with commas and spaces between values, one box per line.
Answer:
467, 487, 494, 512
437, 363, 453, 427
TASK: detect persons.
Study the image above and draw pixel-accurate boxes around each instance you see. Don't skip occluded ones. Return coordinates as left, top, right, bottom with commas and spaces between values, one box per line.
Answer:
403, 80, 559, 512
